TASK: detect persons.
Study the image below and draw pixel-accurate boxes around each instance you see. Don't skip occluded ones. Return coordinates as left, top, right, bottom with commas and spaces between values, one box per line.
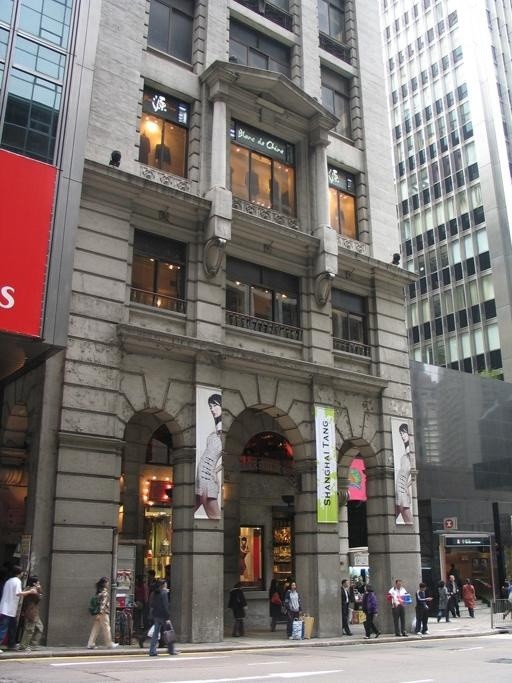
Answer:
195, 393, 222, 521
394, 423, 414, 524
269, 575, 302, 640
437, 563, 512, 624
136, 569, 181, 657
228, 583, 249, 637
238, 536, 250, 580
340, 574, 433, 638
0, 563, 45, 654
86, 576, 120, 650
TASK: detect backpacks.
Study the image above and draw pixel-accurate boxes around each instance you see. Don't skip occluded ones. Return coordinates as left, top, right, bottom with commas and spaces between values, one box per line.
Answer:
88, 595, 101, 617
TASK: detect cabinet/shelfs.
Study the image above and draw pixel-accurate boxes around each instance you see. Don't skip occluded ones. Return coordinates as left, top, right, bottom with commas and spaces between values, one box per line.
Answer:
274, 541, 292, 564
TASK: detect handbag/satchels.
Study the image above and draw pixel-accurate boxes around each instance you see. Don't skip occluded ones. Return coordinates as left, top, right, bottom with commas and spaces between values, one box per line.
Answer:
271, 592, 282, 606
162, 622, 177, 644
291, 612, 316, 640
229, 596, 241, 608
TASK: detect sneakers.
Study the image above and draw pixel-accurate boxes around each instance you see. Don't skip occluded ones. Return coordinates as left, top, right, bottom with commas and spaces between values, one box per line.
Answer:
362, 631, 382, 639
416, 631, 431, 636
88, 640, 181, 656
